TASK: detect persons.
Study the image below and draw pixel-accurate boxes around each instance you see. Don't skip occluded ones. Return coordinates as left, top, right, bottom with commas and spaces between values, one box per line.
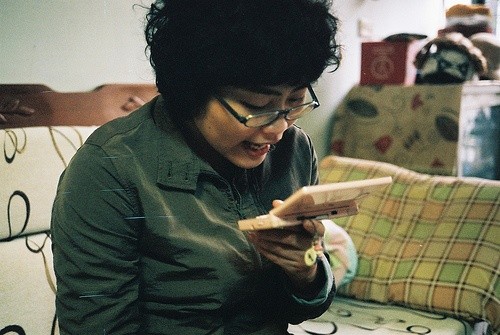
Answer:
51, 1, 343, 335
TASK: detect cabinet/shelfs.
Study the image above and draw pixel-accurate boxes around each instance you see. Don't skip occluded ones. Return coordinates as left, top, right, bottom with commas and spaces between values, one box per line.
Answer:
326, 80, 500, 180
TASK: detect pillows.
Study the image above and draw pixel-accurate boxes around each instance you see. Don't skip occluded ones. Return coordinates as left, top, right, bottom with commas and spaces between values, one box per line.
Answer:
317, 155, 500, 328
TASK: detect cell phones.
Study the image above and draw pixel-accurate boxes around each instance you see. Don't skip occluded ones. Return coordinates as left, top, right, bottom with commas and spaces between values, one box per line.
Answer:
236, 174, 392, 234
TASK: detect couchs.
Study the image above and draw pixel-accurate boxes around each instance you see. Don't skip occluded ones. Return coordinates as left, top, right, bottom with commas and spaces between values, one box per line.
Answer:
0, 82, 489, 335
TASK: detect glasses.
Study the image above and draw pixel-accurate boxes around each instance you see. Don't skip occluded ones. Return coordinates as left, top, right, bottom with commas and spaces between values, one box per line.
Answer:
210, 80, 320, 129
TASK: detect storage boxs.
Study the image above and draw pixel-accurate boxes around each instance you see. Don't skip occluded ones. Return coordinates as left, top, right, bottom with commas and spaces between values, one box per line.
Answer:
360, 33, 430, 86
